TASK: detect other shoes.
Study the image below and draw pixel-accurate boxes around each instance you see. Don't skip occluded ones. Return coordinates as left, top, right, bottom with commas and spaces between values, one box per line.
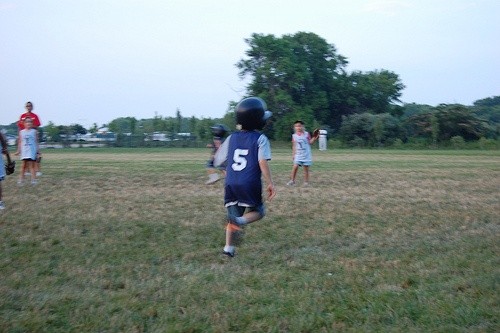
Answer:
227, 215, 243, 247
287, 180, 295, 185
205, 176, 220, 185
223, 247, 234, 257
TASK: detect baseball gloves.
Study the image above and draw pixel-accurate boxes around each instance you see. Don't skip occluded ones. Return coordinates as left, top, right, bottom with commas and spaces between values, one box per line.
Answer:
5, 160, 15, 175
313, 128, 320, 139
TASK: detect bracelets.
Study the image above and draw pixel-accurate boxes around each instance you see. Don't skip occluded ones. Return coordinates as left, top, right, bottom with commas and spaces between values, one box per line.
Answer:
37, 152, 39, 154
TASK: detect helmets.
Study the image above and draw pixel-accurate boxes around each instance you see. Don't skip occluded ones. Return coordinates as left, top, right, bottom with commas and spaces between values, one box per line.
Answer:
235, 97, 272, 130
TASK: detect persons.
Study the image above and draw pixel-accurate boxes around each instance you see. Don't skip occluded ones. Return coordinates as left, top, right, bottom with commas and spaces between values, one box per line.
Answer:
0, 131, 16, 208
15, 116, 38, 184
286, 120, 319, 186
214, 97, 278, 256
16, 101, 41, 176
206, 124, 231, 184
